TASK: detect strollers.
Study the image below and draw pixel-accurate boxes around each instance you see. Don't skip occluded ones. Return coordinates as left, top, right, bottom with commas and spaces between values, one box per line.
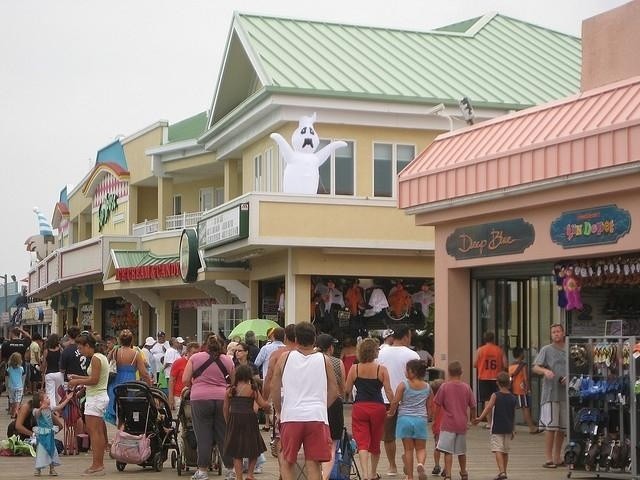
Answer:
169, 389, 223, 478
108, 381, 184, 478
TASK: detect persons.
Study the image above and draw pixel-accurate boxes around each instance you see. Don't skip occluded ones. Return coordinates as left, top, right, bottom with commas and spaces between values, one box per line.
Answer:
1, 322, 540, 478
533, 325, 569, 468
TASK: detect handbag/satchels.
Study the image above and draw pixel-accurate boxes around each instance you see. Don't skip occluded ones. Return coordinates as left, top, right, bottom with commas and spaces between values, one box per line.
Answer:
110, 430, 151, 464
109, 359, 117, 379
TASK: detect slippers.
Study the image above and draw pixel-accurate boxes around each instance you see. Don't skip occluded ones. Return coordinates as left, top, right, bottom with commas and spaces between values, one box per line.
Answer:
565, 342, 640, 468
530, 429, 544, 434
543, 461, 565, 468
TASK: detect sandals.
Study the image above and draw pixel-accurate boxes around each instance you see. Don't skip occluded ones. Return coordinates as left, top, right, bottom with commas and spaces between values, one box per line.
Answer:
80, 464, 106, 475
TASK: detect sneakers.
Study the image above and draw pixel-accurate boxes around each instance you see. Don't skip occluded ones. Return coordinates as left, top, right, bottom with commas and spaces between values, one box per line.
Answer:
50, 470, 57, 476
34, 470, 41, 476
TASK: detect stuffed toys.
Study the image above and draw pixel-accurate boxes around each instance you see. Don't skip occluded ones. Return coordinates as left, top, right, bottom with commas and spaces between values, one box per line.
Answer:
555, 265, 584, 311
270, 111, 349, 197
276, 278, 435, 319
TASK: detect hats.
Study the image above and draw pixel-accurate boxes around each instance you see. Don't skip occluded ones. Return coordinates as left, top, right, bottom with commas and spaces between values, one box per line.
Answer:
177, 337, 185, 343
158, 332, 165, 336
145, 337, 156, 346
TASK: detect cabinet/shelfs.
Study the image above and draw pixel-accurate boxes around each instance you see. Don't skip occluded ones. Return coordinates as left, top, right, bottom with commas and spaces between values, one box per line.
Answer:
563, 334, 640, 480
